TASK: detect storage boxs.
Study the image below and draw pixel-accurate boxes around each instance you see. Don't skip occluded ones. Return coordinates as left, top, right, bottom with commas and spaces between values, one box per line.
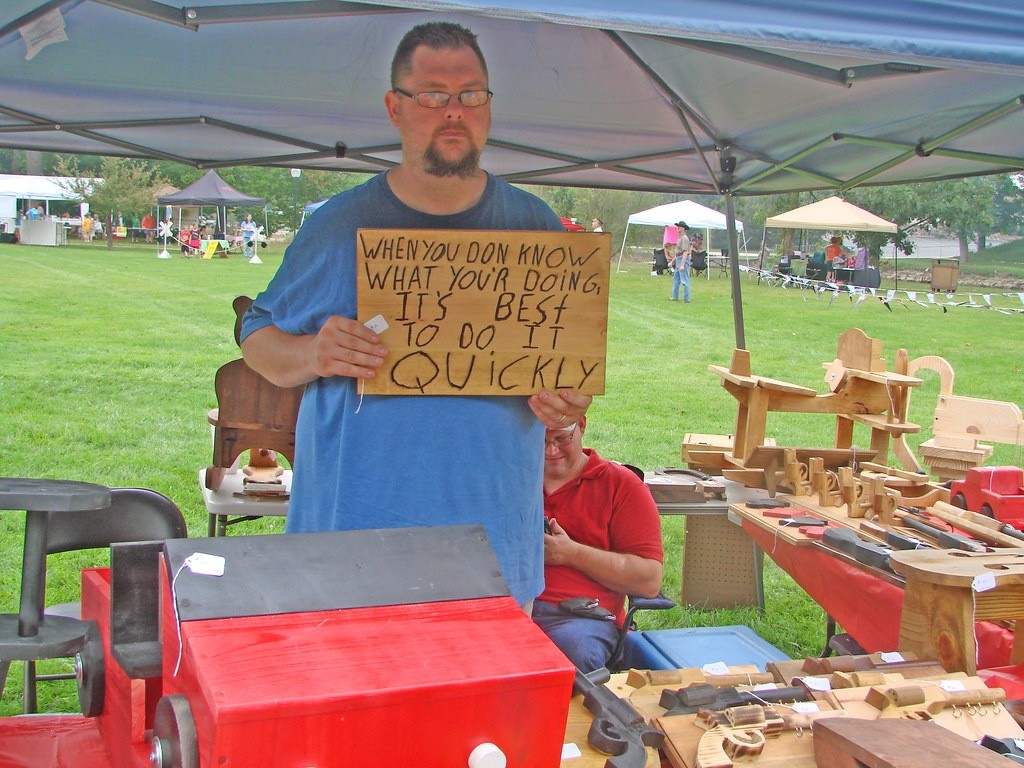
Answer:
623, 626, 792, 671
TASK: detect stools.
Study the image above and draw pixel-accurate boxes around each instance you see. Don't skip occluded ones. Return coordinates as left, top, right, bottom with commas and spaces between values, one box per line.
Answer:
830, 634, 868, 657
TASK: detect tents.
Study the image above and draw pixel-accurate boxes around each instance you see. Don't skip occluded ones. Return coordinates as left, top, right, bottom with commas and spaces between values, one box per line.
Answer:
300, 199, 330, 228
757, 195, 898, 291
617, 198, 752, 280
157, 168, 268, 254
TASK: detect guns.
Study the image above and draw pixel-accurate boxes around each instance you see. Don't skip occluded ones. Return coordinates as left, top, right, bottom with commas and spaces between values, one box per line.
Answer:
697, 703, 847, 768
572, 667, 664, 768
659, 684, 804, 716
866, 682, 1007, 722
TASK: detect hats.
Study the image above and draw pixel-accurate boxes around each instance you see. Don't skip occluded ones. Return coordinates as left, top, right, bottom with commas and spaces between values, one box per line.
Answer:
675, 221, 690, 230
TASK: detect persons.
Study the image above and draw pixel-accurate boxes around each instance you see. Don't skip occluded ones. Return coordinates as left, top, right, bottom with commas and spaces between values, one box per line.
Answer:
591, 217, 604, 233
664, 221, 702, 303
236, 18, 592, 620
25, 202, 257, 259
823, 236, 852, 282
531, 414, 663, 675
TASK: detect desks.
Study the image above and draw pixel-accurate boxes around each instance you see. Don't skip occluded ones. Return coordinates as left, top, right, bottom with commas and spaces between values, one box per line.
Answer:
833, 267, 862, 284
200, 240, 229, 257
651, 475, 791, 515
198, 467, 293, 537
705, 256, 729, 280
126, 228, 158, 243
227, 235, 244, 254
727, 507, 1015, 671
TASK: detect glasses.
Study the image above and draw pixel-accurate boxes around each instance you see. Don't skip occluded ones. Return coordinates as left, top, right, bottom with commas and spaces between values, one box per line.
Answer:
545, 424, 577, 448
394, 87, 493, 108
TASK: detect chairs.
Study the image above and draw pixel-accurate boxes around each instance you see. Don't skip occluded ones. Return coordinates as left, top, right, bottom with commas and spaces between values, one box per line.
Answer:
652, 248, 728, 279
204, 296, 306, 492
786, 259, 807, 278
22, 487, 187, 714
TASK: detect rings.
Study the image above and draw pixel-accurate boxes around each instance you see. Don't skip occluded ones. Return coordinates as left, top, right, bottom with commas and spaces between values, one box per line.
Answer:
555, 414, 566, 423
